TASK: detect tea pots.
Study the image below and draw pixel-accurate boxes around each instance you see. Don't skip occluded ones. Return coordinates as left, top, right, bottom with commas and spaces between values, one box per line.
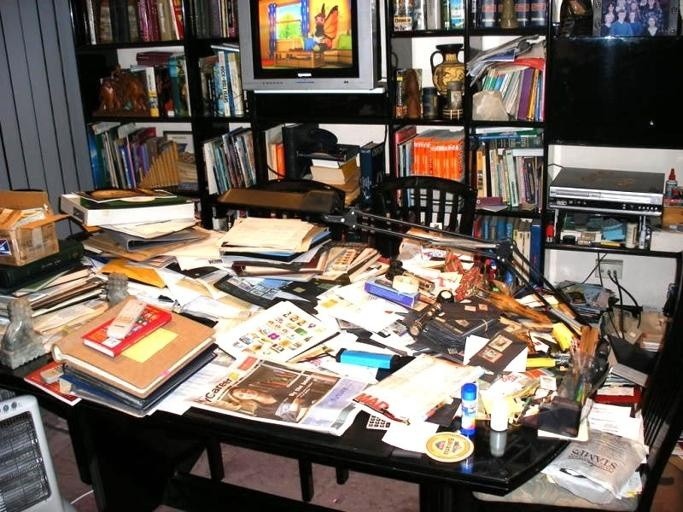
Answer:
430, 43, 465, 120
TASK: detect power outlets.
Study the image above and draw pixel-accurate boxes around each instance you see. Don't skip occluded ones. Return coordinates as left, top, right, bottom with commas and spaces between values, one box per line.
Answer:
595, 260, 622, 281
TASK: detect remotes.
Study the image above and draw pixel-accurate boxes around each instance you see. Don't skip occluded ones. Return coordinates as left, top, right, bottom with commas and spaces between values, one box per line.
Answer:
40, 365, 64, 385
106, 300, 147, 340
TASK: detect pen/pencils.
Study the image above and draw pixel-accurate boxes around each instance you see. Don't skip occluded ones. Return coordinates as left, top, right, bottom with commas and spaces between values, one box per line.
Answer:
515, 397, 533, 423
567, 336, 590, 405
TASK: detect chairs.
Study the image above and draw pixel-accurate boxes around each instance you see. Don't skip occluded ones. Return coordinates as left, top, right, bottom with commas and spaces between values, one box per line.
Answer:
421, 291, 682, 511
91, 439, 175, 512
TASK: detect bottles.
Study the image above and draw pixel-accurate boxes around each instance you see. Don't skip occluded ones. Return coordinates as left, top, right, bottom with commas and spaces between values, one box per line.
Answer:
576, 233, 596, 246
500, 1, 519, 29
624, 219, 650, 249
665, 168, 677, 206
460, 383, 478, 435
460, 434, 474, 474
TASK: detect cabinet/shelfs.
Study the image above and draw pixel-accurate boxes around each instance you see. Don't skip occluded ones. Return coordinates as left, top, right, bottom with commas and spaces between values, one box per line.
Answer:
387, 2, 471, 246
469, 1, 549, 288
71, 1, 251, 245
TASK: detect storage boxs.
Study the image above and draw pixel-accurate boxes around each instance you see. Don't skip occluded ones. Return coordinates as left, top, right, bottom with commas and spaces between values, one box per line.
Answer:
0, 189, 71, 270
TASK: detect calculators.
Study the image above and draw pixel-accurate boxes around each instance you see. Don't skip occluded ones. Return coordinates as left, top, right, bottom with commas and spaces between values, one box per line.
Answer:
366, 414, 390, 431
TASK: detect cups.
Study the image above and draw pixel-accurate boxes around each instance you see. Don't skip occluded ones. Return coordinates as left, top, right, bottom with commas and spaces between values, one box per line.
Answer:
488, 429, 506, 456
490, 401, 509, 431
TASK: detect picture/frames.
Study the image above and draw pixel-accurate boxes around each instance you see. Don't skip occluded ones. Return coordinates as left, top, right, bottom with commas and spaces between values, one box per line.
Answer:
254, 0, 361, 78
592, 2, 678, 36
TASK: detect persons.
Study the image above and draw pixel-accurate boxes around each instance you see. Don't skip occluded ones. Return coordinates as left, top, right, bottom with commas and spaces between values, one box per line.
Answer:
599, 0, 663, 35
229, 384, 318, 422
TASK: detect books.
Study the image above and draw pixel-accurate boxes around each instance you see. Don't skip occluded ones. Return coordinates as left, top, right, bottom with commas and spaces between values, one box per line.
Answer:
92, 123, 256, 197
57, 188, 500, 285
79, 0, 249, 118
0, 285, 221, 415
393, 1, 545, 123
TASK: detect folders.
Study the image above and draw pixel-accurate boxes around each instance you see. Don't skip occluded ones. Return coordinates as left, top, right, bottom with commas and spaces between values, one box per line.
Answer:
233, 246, 328, 276
102, 226, 210, 252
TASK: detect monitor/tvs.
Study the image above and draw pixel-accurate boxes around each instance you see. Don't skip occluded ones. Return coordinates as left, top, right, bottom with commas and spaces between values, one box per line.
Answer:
238, 0, 380, 91
